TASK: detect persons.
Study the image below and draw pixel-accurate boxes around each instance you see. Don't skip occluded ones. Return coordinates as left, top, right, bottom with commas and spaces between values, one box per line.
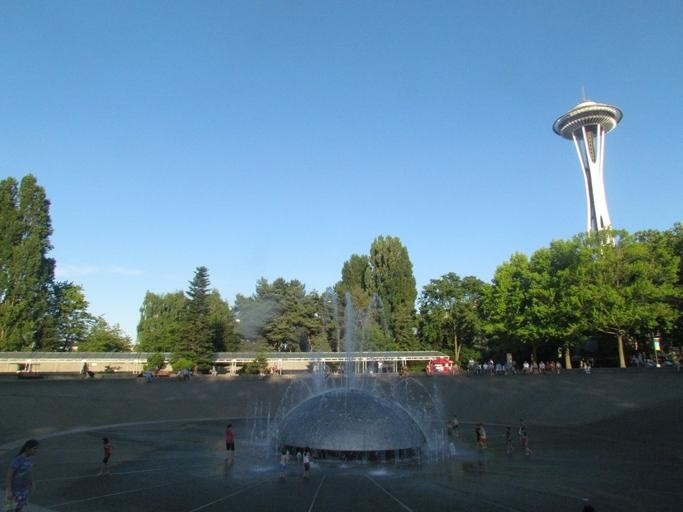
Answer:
97, 436, 113, 476
402, 363, 409, 378
398, 364, 403, 378
582, 358, 593, 376
145, 365, 196, 384
629, 350, 683, 373
466, 357, 562, 377
312, 362, 345, 376
224, 423, 235, 462
302, 446, 311, 481
580, 497, 595, 512
445, 414, 533, 456
5, 438, 41, 512
259, 364, 278, 376
80, 362, 89, 380
378, 361, 384, 373
279, 448, 288, 479
